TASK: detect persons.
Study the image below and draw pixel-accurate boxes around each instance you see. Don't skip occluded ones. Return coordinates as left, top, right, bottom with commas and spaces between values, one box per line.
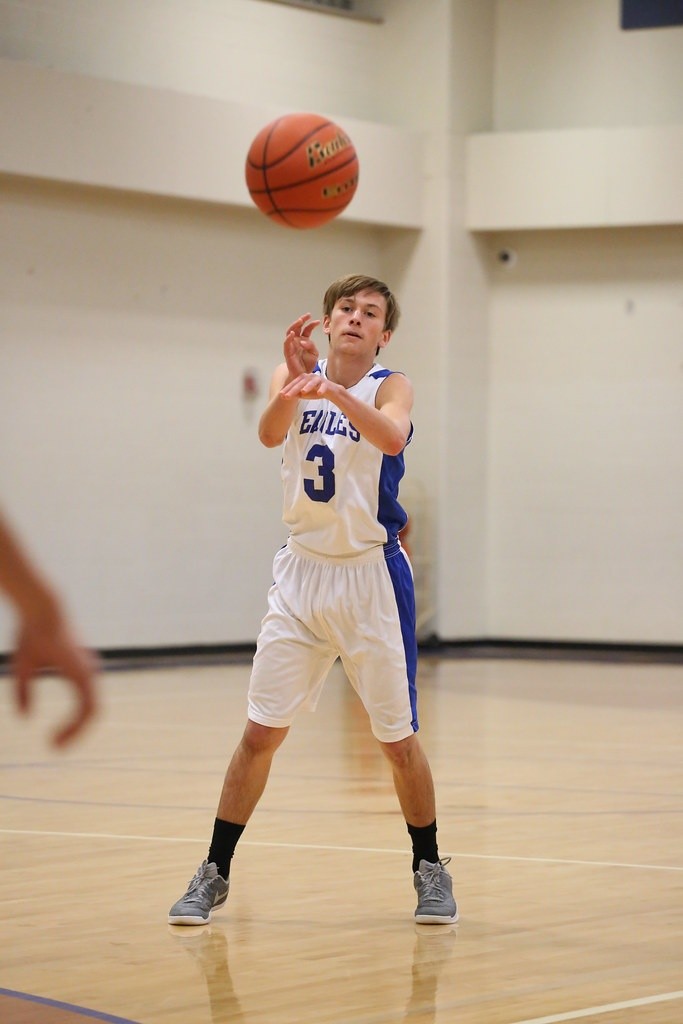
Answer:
0, 513, 100, 747
169, 275, 462, 927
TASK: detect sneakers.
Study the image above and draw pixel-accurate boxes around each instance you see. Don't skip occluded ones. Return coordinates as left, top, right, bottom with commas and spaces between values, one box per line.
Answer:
169, 860, 230, 925
413, 859, 458, 924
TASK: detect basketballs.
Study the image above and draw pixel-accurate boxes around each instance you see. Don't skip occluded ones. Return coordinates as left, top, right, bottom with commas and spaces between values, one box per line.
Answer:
245, 110, 358, 230
